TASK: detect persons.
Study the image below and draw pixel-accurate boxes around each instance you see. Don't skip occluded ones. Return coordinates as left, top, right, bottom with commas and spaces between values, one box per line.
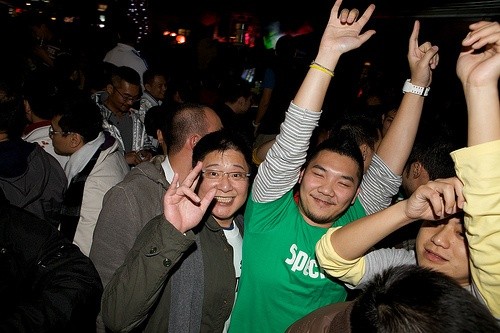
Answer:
228, 0, 440, 333
313, 17, 500, 327
0, 10, 460, 333
285, 266, 500, 333
100, 127, 250, 333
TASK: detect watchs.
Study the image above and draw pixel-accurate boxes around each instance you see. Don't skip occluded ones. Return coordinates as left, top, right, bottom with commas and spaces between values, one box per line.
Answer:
401, 78, 430, 97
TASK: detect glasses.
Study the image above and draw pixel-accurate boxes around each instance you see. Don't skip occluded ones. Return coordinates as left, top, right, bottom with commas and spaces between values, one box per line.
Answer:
48, 125, 84, 140
199, 169, 251, 182
111, 83, 141, 102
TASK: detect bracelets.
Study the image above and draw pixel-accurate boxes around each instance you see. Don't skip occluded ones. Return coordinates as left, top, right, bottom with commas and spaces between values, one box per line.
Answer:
307, 61, 335, 77
